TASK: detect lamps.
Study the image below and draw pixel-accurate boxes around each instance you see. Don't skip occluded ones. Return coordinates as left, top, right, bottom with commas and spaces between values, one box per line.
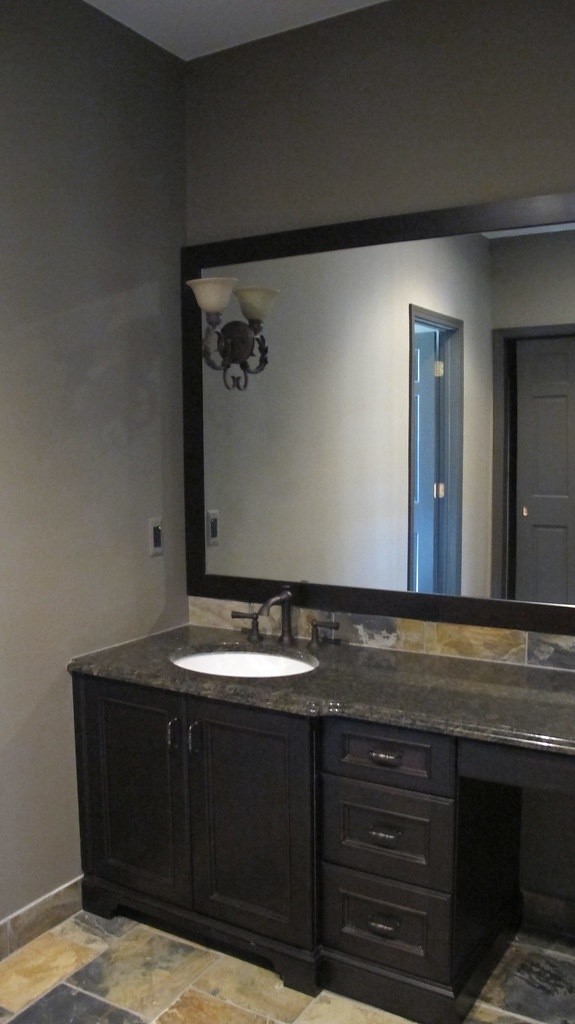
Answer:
188, 276, 253, 391
225, 287, 281, 392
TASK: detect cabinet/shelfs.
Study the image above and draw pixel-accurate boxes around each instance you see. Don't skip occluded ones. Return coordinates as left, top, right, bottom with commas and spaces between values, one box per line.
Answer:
72, 670, 524, 1024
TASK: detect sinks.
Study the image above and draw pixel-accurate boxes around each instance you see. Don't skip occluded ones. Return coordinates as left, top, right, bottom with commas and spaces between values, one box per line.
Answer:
169, 642, 318, 678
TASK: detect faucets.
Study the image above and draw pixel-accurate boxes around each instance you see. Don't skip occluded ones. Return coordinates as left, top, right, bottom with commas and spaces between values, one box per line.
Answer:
258, 584, 294, 644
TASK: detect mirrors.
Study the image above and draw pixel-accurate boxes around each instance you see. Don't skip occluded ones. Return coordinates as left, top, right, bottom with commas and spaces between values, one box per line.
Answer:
181, 193, 575, 636
